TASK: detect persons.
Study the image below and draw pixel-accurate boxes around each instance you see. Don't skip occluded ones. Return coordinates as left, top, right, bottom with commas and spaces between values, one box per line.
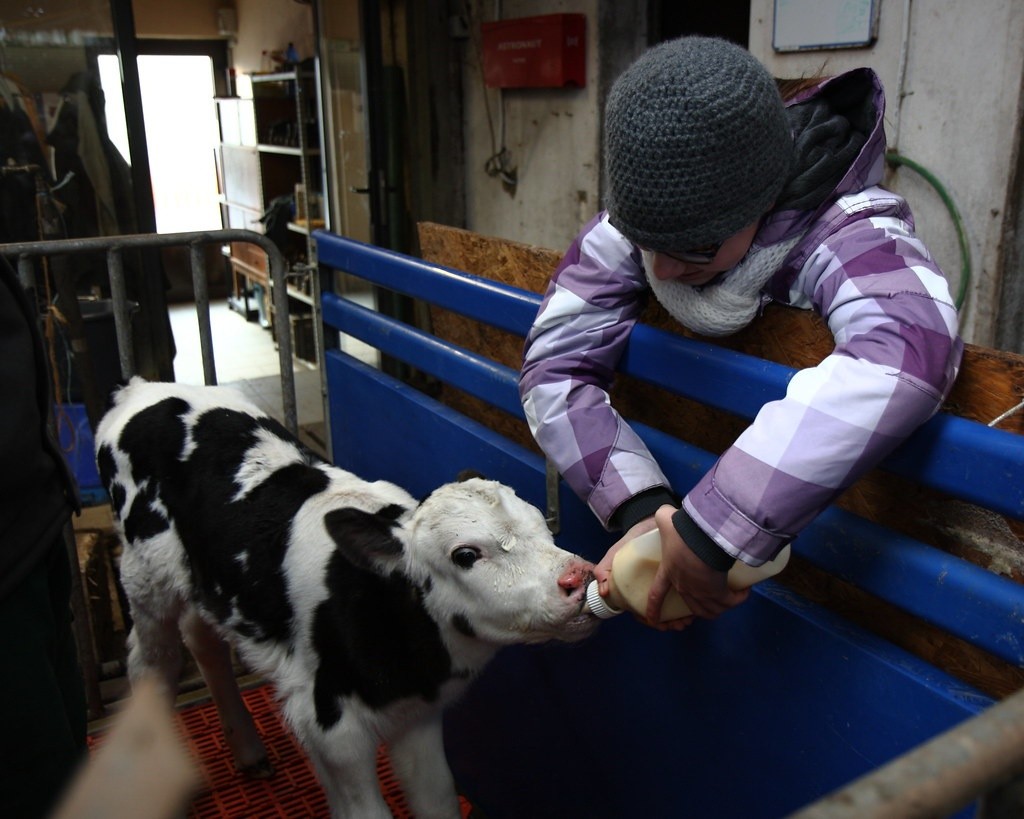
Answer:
517, 36, 966, 628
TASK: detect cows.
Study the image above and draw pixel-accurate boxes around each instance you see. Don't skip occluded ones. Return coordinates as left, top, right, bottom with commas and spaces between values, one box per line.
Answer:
93, 368, 614, 819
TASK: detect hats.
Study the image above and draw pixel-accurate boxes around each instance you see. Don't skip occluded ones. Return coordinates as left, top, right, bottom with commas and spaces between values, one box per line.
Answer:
603, 36, 791, 249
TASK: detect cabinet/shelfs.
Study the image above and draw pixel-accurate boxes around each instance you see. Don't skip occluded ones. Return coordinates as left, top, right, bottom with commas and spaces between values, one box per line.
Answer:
212, 68, 325, 371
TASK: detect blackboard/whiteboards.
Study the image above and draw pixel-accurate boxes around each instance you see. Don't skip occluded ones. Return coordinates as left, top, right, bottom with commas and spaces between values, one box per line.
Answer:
770, 0, 882, 54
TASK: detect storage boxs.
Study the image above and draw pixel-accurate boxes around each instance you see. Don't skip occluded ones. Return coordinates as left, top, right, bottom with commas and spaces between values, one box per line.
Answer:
269, 304, 315, 358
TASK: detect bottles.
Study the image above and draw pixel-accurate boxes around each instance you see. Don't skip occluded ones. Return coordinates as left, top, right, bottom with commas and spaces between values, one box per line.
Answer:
580, 527, 790, 623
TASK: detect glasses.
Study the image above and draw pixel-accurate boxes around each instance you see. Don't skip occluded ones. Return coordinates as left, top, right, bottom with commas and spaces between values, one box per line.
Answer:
629, 240, 723, 264
